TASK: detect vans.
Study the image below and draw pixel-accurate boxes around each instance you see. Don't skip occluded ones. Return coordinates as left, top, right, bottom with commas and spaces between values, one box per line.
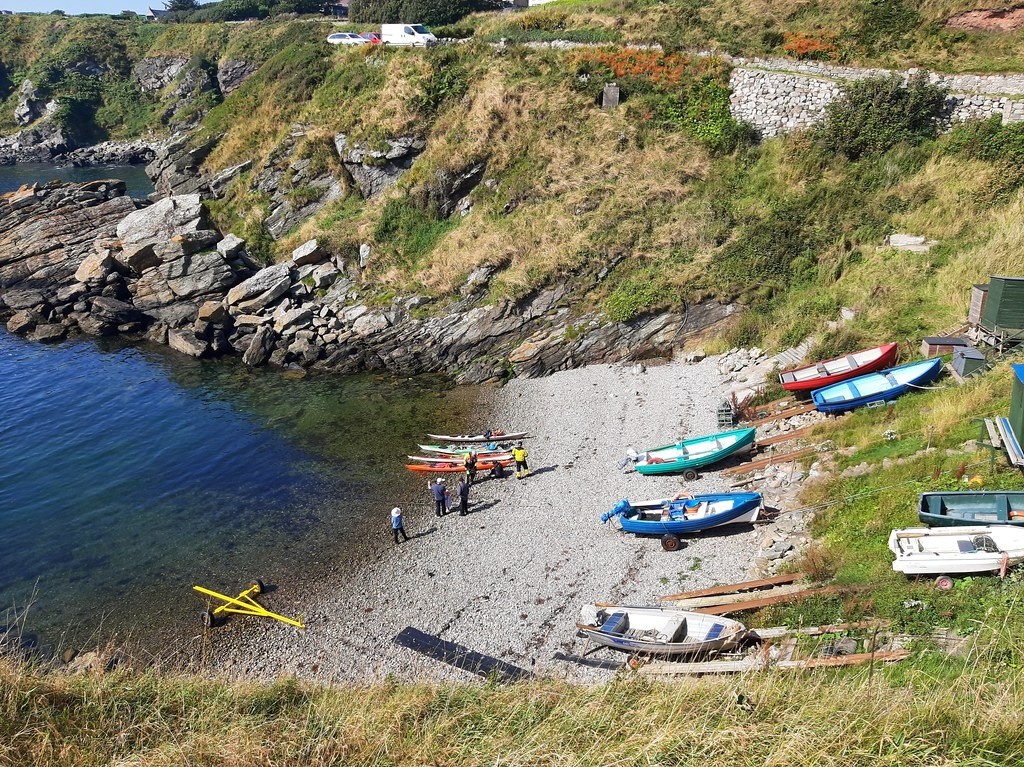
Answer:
381, 24, 438, 43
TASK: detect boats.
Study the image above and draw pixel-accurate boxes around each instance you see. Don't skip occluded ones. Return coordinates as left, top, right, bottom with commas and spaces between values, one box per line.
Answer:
416, 442, 517, 455
811, 358, 941, 414
406, 454, 514, 463
620, 492, 761, 533
779, 340, 897, 391
577, 603, 747, 655
634, 426, 755, 475
427, 432, 529, 441
918, 491, 1024, 527
887, 524, 1024, 574
404, 460, 514, 472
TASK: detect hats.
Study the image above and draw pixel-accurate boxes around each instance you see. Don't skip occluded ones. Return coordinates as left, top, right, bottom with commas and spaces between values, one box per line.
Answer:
390, 506, 402, 517
492, 460, 497, 464
518, 441, 522, 445
437, 477, 446, 483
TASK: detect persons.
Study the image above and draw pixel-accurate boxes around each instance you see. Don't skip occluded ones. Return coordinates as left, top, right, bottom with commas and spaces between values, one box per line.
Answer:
428, 478, 447, 518
488, 460, 503, 478
391, 507, 408, 544
511, 441, 529, 480
464, 449, 478, 484
457, 478, 469, 516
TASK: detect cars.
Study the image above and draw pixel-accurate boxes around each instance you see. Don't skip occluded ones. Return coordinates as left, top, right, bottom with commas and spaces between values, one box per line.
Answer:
358, 32, 382, 45
327, 32, 372, 45
